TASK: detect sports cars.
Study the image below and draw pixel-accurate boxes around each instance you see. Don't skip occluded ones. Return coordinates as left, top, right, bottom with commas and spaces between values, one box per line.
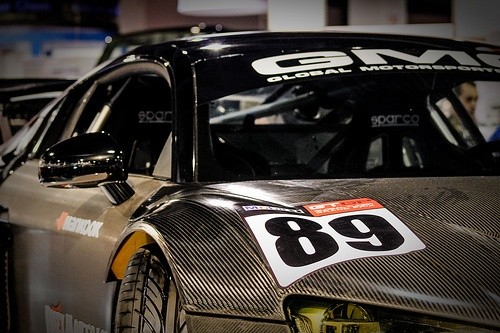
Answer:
1, 30, 500, 333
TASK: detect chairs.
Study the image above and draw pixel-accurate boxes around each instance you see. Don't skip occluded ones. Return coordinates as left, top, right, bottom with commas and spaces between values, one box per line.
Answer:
212, 126, 290, 182
310, 123, 384, 181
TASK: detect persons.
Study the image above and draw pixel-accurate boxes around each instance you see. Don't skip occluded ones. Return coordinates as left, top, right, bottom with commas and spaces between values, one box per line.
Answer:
432, 82, 484, 146
